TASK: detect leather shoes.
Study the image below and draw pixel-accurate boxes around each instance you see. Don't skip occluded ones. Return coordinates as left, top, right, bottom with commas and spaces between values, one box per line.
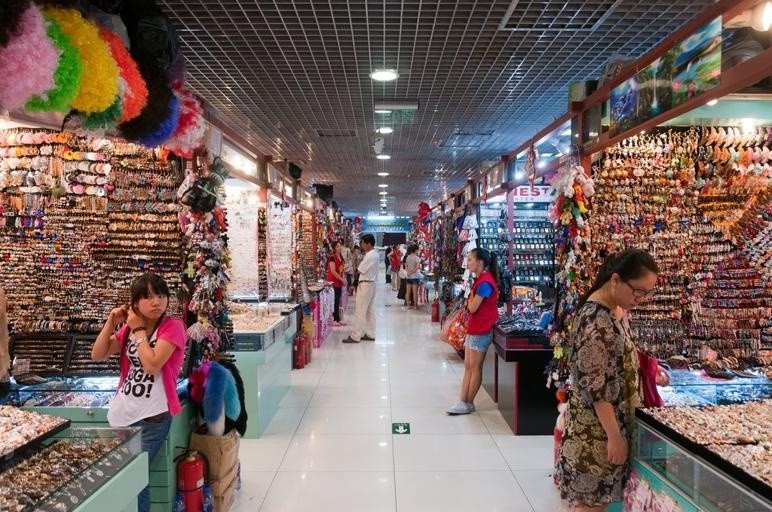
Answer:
361, 334, 375, 340
342, 336, 360, 343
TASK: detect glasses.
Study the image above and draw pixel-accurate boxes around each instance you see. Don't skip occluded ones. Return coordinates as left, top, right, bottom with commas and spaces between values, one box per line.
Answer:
625, 281, 657, 298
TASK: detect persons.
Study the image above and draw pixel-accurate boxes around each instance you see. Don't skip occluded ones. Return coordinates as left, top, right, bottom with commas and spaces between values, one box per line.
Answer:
340, 237, 425, 310
445, 247, 501, 415
342, 232, 380, 345
550, 245, 676, 511
325, 240, 350, 327
90, 272, 188, 512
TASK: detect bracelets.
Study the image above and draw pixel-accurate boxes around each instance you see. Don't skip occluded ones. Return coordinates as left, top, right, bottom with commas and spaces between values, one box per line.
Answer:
24, 320, 105, 334
132, 336, 148, 348
131, 325, 146, 333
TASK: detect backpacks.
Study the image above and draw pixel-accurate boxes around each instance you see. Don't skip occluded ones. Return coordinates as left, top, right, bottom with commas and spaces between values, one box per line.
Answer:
492, 251, 512, 302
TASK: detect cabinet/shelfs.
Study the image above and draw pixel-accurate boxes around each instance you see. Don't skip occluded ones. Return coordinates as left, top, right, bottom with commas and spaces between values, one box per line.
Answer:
478, 330, 562, 438
618, 366, 705, 512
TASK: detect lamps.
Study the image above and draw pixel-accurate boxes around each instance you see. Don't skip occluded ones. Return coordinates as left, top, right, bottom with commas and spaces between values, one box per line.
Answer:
374, 98, 419, 110
369, 68, 399, 82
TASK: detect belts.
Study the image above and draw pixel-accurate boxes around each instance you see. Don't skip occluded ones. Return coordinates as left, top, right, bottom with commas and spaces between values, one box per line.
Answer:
358, 280, 373, 283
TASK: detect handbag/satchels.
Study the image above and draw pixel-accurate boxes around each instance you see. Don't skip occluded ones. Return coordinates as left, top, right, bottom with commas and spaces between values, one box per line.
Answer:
456, 215, 479, 281
636, 350, 664, 408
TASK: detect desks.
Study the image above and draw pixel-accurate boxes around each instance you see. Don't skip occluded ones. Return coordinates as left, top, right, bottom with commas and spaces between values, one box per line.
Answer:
225, 332, 292, 440
23, 404, 197, 512
71, 453, 151, 512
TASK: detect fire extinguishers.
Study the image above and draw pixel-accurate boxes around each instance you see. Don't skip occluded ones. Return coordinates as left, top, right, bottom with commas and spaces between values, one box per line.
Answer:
293, 330, 311, 369
173, 446, 210, 512
441, 310, 450, 330
432, 298, 439, 322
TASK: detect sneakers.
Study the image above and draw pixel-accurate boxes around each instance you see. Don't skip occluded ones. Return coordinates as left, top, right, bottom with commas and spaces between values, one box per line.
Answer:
333, 321, 347, 325
445, 400, 475, 414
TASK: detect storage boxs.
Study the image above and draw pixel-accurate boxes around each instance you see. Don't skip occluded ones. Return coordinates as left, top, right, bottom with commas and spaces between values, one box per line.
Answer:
189, 429, 241, 512
